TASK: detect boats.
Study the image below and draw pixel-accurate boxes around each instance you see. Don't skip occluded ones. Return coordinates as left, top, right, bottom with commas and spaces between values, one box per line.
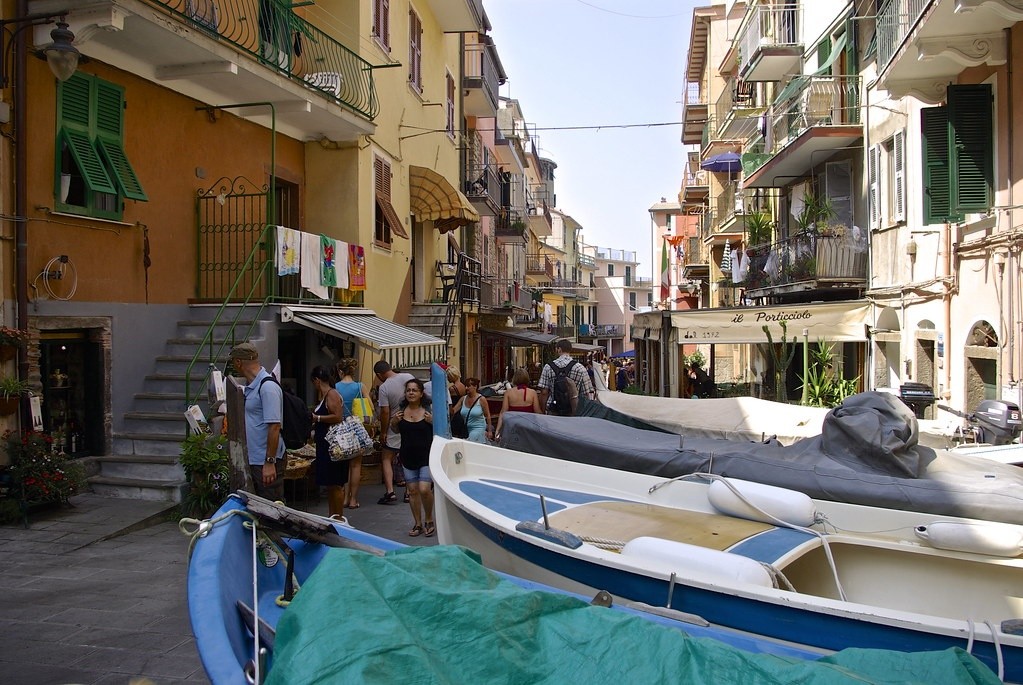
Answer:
429, 360, 1023, 685
186, 489, 1015, 685
499, 382, 1023, 526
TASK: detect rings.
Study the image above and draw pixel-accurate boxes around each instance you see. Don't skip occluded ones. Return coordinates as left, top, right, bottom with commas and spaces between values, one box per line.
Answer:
424, 414, 426, 417
399, 414, 401, 417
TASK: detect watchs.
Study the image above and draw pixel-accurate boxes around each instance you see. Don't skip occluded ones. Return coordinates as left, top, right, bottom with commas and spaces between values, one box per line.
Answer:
266, 458, 277, 464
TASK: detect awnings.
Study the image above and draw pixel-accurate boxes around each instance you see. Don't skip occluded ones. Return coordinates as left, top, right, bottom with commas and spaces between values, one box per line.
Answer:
609, 349, 636, 358
775, 80, 811, 106
408, 164, 480, 232
789, 31, 846, 99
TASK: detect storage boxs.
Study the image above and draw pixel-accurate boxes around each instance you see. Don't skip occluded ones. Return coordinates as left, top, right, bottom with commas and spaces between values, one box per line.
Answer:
285, 466, 309, 479
359, 422, 382, 486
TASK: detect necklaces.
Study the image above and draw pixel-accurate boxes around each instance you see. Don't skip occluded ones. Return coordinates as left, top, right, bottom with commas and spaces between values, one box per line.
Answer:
406, 409, 420, 418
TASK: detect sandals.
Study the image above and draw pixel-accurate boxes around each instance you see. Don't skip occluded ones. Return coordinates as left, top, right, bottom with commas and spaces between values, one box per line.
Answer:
403, 491, 410, 503
377, 491, 399, 504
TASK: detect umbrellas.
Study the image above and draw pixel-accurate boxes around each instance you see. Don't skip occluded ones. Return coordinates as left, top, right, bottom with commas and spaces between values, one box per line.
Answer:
720, 239, 732, 276
701, 151, 743, 187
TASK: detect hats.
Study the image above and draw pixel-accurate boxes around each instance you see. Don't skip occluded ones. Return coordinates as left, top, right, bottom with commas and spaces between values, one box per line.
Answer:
230, 342, 260, 361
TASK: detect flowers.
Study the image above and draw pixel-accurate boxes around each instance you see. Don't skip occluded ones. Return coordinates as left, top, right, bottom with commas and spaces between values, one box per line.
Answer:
178, 431, 230, 514
50, 369, 68, 380
0, 324, 29, 350
0, 427, 67, 504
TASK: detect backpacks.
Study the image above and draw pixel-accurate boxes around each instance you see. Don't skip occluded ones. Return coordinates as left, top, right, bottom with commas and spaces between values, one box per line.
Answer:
546, 359, 580, 410
258, 376, 314, 450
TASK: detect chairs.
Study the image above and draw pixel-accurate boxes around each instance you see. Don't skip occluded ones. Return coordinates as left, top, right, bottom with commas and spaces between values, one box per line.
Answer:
790, 78, 836, 133
438, 261, 460, 303
304, 72, 342, 104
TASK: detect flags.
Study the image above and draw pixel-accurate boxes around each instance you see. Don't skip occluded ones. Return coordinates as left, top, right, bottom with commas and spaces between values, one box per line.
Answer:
660, 241, 671, 303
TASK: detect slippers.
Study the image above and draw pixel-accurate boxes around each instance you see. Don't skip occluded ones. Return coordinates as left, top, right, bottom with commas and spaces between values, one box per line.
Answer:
408, 524, 425, 538
425, 521, 437, 537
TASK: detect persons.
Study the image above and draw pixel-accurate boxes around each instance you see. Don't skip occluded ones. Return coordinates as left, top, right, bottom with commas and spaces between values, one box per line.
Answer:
684, 362, 715, 398
495, 368, 542, 439
587, 349, 636, 393
310, 368, 349, 521
231, 343, 288, 503
390, 378, 436, 537
334, 358, 375, 508
423, 362, 492, 443
374, 360, 415, 505
538, 339, 594, 417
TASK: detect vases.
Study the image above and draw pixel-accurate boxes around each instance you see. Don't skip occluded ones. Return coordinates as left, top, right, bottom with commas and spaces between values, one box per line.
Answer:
51, 379, 63, 386
20, 504, 64, 522
0, 345, 17, 359
0, 472, 11, 488
191, 471, 208, 494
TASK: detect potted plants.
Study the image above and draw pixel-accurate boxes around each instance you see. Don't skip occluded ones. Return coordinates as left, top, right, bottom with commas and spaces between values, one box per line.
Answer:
0, 372, 35, 416
745, 189, 847, 289
512, 222, 527, 229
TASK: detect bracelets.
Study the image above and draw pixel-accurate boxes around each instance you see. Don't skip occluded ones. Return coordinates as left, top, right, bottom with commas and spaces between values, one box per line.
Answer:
317, 414, 321, 424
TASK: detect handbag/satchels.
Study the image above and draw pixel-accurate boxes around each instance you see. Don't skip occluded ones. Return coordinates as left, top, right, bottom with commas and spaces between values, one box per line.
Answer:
352, 380, 374, 432
460, 423, 469, 439
324, 415, 375, 463
